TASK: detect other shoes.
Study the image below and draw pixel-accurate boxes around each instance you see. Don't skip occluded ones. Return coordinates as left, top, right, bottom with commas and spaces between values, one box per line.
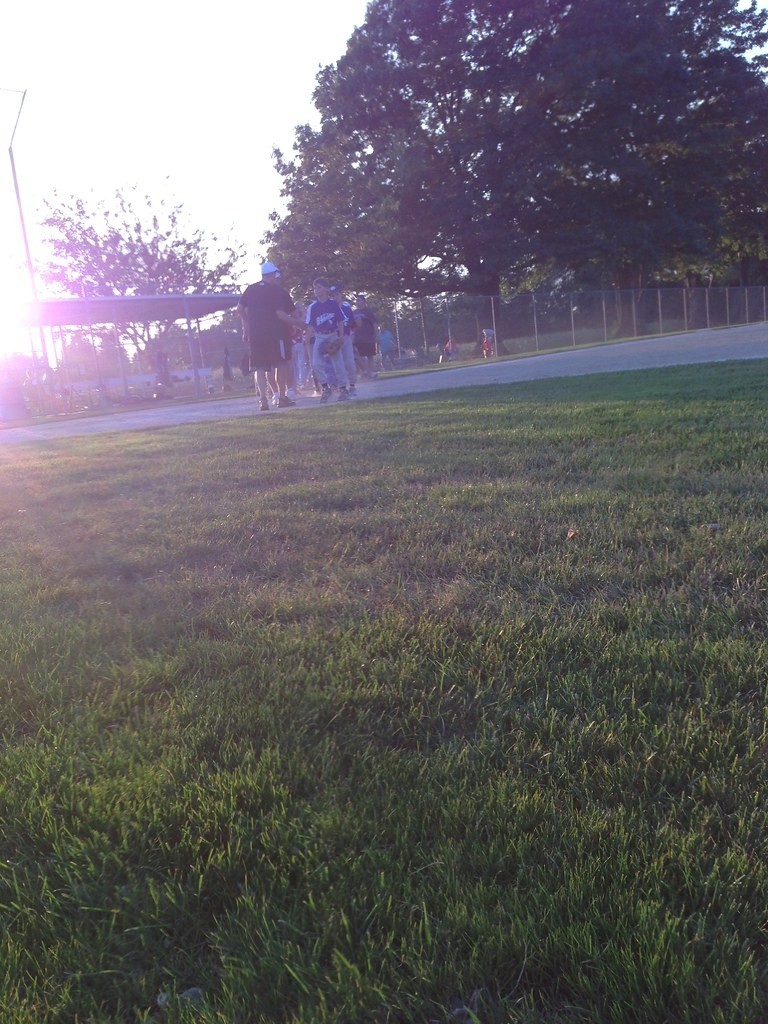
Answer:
259, 402, 269, 410
349, 387, 356, 396
320, 388, 331, 403
271, 397, 277, 405
337, 390, 349, 401
285, 391, 297, 400
369, 372, 378, 378
256, 390, 260, 397
267, 389, 273, 399
277, 395, 296, 407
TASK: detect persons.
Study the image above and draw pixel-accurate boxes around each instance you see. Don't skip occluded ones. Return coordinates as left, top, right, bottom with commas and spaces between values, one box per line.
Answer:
375, 322, 398, 372
436, 336, 458, 363
481, 328, 496, 359
238, 263, 379, 412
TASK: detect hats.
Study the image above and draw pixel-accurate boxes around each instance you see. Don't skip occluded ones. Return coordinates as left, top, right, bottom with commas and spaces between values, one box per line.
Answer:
261, 262, 276, 274
291, 287, 305, 301
329, 285, 337, 292
356, 295, 365, 301
